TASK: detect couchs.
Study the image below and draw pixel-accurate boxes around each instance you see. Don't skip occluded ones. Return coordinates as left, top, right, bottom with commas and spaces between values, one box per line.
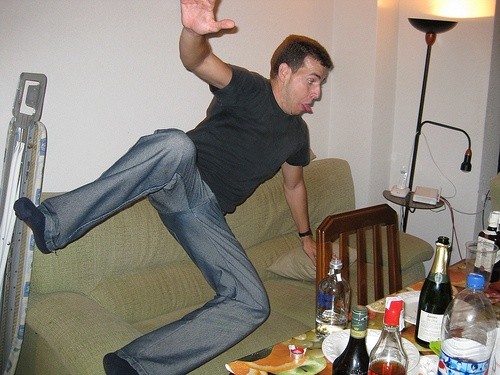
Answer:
0, 157, 434, 375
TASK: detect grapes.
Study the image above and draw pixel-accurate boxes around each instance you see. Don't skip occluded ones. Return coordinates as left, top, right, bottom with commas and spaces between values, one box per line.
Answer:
281, 330, 324, 358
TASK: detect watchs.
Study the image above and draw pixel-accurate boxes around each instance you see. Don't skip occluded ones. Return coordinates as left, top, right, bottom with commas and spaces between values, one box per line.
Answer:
298, 230, 312, 237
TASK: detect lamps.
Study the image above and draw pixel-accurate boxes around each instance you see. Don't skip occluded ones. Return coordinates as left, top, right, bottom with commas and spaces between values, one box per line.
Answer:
382, 17, 472, 234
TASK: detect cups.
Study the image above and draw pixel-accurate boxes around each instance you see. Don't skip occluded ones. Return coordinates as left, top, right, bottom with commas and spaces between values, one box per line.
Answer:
465, 241, 499, 293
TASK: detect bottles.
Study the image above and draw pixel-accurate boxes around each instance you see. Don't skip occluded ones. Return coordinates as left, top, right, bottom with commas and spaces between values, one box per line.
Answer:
473, 211, 500, 282
368, 296, 408, 375
314, 257, 351, 342
332, 306, 370, 375
414, 236, 453, 350
437, 273, 498, 375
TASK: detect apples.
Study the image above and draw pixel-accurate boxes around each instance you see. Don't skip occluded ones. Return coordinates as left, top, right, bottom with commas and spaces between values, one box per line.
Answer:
272, 357, 326, 375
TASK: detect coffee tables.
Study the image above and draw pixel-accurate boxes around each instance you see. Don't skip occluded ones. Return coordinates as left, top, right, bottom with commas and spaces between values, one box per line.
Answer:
224, 255, 500, 375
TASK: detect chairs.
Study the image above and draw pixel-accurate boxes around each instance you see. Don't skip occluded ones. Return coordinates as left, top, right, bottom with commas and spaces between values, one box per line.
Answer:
314, 202, 403, 324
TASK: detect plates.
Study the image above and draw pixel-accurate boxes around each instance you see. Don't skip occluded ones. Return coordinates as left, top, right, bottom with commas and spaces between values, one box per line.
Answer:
408, 355, 440, 375
321, 329, 420, 373
394, 290, 477, 330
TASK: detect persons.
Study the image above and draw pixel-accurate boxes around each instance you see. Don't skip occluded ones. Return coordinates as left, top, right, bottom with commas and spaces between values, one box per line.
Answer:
13, 0, 336, 375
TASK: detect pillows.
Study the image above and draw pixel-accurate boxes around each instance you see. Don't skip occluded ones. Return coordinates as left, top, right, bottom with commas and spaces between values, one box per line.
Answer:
264, 241, 357, 281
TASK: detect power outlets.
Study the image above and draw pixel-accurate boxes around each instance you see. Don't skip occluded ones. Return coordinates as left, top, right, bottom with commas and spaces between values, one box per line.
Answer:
481, 193, 492, 203
